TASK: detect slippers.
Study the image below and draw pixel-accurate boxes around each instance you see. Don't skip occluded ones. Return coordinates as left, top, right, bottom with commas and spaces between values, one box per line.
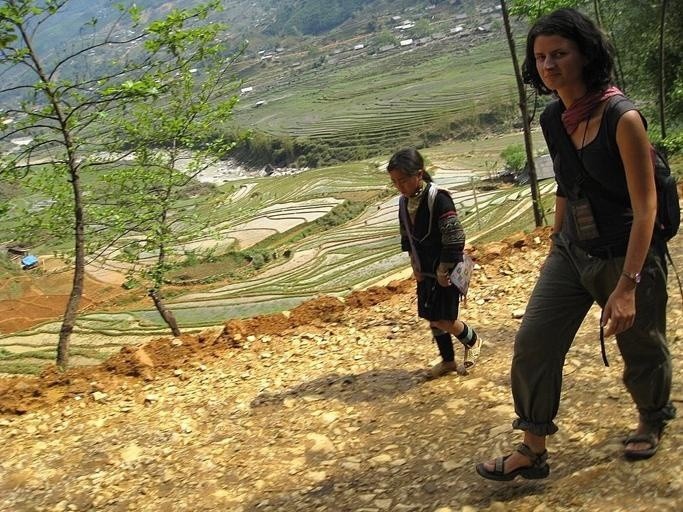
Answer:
426, 361, 458, 378
464, 338, 482, 370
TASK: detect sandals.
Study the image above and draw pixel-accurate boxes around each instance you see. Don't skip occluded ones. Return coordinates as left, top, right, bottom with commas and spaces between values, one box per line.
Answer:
475, 442, 551, 482
622, 420, 668, 459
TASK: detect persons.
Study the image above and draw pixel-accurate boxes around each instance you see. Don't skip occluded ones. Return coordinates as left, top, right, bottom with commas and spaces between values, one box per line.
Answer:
473, 10, 677, 480
385, 147, 482, 380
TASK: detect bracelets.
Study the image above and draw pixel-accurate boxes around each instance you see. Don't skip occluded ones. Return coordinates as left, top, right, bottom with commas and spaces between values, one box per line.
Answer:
436, 270, 450, 276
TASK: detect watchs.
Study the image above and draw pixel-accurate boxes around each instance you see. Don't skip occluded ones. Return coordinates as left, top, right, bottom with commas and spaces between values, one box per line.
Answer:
620, 271, 643, 284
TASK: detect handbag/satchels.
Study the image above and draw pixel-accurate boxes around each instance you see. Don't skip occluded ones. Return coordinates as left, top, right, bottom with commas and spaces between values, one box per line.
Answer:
449, 253, 474, 298
652, 151, 680, 244
